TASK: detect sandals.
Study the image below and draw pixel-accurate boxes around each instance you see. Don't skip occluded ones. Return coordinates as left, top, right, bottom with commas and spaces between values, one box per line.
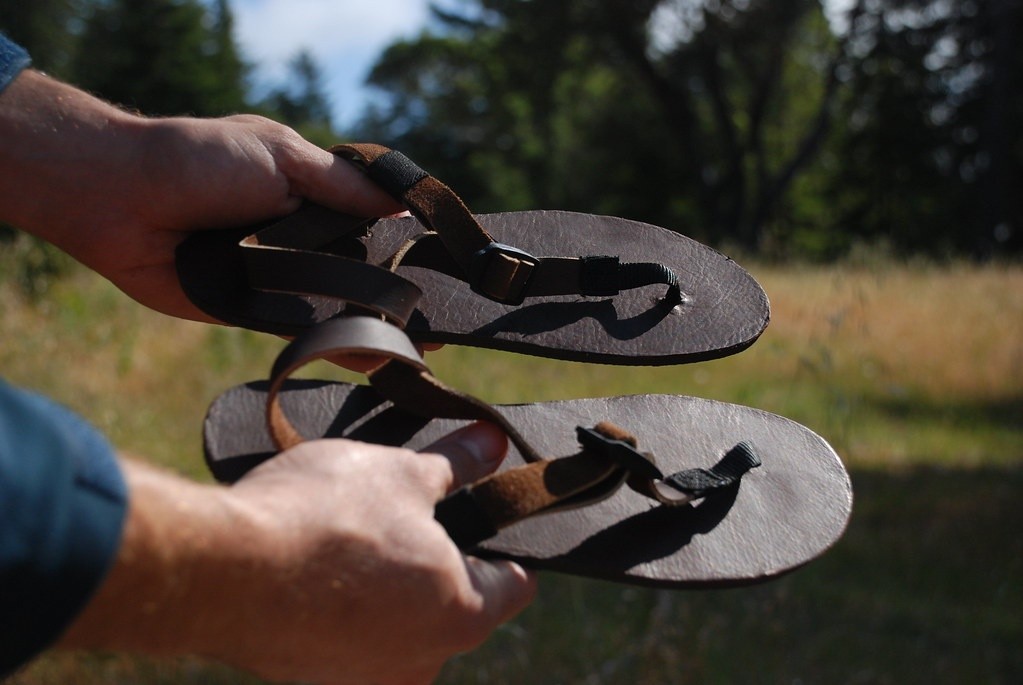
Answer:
202, 316, 855, 588
174, 143, 771, 369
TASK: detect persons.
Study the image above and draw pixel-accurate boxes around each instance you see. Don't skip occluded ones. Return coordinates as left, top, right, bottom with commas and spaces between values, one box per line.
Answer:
1, 36, 538, 684
